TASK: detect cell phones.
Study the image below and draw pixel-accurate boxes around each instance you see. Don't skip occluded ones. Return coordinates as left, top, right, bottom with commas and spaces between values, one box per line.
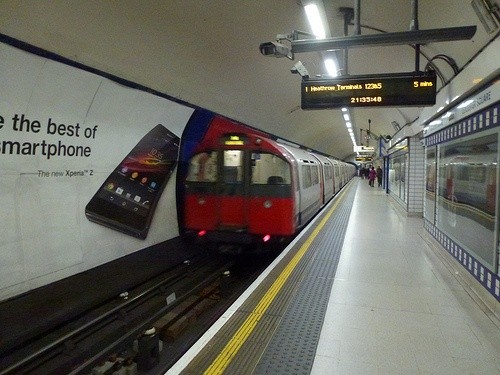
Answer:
85, 123, 181, 239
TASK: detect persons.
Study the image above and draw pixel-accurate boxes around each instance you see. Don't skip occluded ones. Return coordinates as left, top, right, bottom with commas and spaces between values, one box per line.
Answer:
357, 166, 371, 179
368, 166, 382, 187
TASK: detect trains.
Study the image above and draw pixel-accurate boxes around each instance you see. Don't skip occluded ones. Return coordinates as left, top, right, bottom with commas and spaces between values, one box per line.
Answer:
183, 131, 360, 249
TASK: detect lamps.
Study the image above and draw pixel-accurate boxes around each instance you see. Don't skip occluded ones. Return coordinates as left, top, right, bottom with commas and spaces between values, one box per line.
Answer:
302, 0, 357, 147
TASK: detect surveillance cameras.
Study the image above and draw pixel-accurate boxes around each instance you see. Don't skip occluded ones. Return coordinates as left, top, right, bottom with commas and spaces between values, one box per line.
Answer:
291, 61, 310, 82
259, 36, 294, 59
